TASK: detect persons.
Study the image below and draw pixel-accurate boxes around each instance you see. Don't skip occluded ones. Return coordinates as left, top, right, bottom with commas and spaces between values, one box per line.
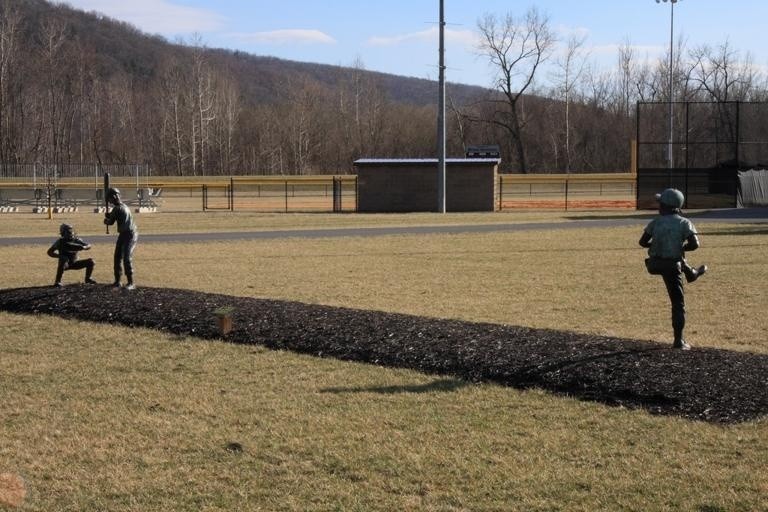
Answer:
104, 187, 139, 290
639, 189, 707, 350
47, 224, 96, 287
